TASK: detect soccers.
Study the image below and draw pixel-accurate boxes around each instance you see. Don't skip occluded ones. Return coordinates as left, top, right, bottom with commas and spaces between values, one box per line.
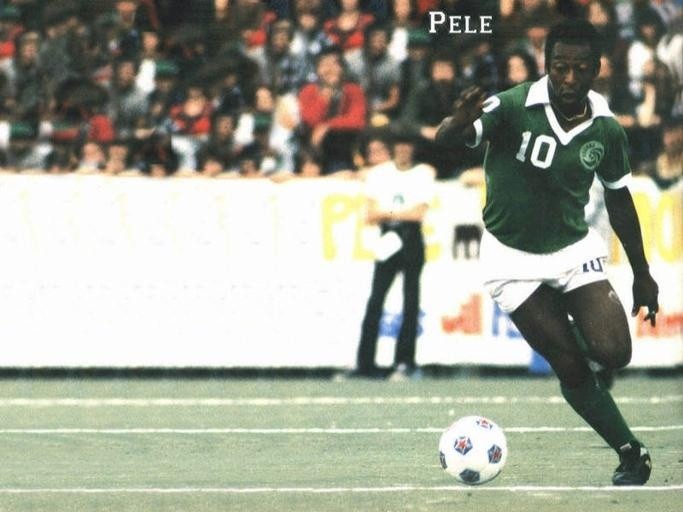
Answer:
439, 416, 506, 484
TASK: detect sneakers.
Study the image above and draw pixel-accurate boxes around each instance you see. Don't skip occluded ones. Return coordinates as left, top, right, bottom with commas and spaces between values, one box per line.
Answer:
335, 362, 414, 382
612, 440, 651, 484
572, 321, 612, 387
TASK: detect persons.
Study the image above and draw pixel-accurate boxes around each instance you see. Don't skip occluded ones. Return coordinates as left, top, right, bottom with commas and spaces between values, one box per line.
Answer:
335, 116, 438, 387
0, 1, 682, 193
430, 13, 663, 484
